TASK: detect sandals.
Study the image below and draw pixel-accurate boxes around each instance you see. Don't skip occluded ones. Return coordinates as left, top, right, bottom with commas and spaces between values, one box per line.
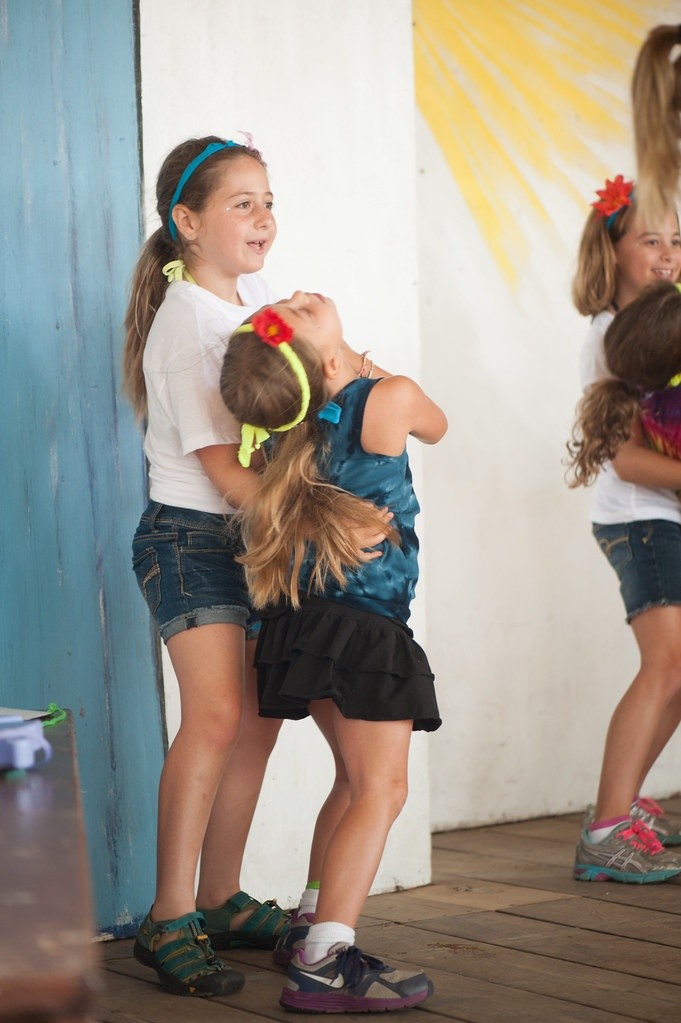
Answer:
133, 904, 245, 997
197, 891, 294, 950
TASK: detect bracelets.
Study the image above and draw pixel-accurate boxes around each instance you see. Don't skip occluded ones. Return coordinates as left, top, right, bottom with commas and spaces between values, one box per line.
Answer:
357, 350, 373, 379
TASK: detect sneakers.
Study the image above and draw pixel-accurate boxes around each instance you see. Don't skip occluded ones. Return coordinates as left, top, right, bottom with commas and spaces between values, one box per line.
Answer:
573, 821, 681, 883
582, 795, 681, 847
279, 942, 434, 1013
272, 909, 315, 967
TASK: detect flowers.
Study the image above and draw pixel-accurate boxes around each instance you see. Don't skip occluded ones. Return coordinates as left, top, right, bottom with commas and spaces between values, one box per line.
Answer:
592, 173, 635, 223
252, 305, 294, 348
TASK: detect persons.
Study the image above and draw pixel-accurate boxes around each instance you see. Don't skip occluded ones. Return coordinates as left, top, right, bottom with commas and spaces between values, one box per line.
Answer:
566, 24, 681, 884
122, 138, 291, 998
219, 289, 447, 1014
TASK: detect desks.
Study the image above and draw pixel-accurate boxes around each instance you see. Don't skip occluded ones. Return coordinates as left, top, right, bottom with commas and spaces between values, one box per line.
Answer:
0, 705, 105, 1023
